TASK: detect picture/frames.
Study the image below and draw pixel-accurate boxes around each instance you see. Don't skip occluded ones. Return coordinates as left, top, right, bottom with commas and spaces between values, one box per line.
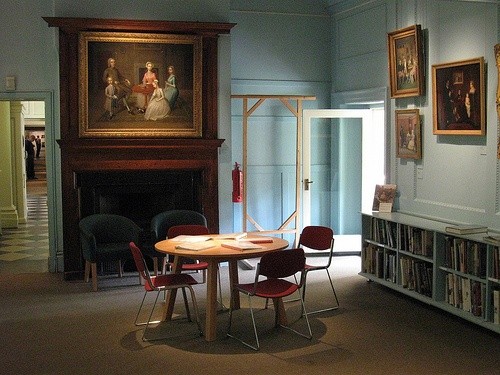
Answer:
385, 25, 426, 99
430, 57, 487, 136
77, 31, 203, 138
394, 109, 422, 159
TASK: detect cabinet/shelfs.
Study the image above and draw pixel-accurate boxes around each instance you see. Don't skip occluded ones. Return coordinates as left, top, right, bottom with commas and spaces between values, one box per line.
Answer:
356, 211, 500, 333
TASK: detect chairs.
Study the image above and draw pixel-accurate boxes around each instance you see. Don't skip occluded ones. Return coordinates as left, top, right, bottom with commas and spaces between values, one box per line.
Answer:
79, 211, 342, 350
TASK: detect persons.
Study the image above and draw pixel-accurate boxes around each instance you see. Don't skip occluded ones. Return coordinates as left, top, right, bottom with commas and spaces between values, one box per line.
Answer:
24, 132, 41, 179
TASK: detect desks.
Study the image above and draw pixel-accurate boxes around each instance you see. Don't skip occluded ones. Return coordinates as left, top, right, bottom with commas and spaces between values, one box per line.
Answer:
155, 233, 289, 341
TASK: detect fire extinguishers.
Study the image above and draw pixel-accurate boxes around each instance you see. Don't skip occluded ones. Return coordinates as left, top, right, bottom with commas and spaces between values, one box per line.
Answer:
232, 161, 243, 202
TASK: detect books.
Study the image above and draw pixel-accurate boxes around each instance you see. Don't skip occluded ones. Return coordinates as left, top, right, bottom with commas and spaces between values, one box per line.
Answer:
240, 237, 273, 243
171, 235, 212, 242
444, 273, 500, 325
445, 225, 488, 235
483, 235, 500, 245
369, 217, 433, 257
441, 235, 500, 280
216, 233, 247, 240
361, 243, 433, 297
221, 241, 263, 252
175, 241, 216, 251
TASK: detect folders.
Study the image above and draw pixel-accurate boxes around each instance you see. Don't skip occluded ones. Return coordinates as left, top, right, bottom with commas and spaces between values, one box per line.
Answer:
172, 235, 213, 241
221, 241, 262, 251
237, 238, 273, 243
213, 232, 247, 241
175, 241, 216, 251
